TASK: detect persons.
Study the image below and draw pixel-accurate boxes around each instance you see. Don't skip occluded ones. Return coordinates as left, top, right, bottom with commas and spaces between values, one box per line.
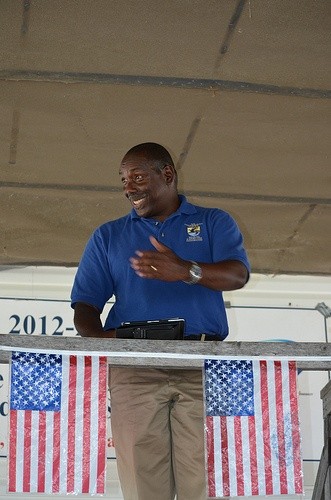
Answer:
70, 142, 251, 499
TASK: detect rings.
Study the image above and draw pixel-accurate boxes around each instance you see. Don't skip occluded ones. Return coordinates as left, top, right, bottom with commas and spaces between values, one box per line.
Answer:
151, 266, 158, 272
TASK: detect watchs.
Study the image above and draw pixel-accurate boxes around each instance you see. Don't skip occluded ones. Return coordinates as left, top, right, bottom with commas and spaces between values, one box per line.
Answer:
184, 260, 203, 286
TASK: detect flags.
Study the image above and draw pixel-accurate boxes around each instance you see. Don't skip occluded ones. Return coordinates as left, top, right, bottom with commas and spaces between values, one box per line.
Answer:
7, 351, 110, 496
201, 358, 305, 497
